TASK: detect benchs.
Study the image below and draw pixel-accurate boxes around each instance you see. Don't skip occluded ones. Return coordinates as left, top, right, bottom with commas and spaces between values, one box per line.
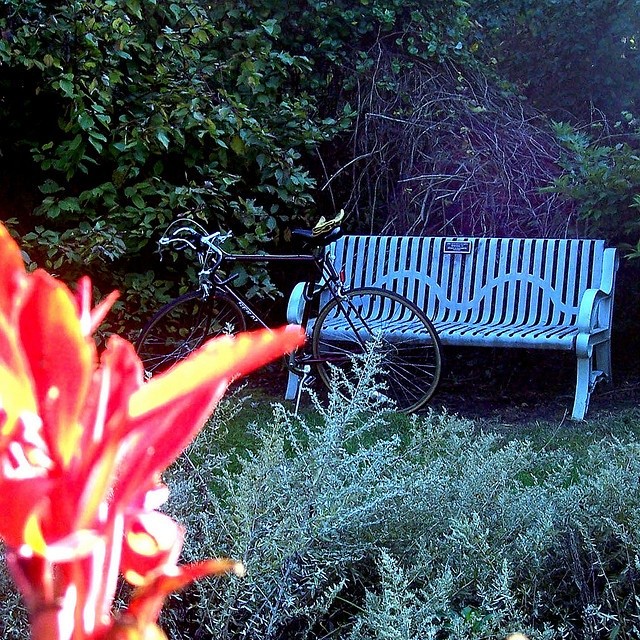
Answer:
284, 234, 621, 423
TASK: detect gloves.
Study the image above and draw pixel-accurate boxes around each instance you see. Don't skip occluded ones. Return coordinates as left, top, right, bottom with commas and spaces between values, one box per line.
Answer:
310, 209, 345, 238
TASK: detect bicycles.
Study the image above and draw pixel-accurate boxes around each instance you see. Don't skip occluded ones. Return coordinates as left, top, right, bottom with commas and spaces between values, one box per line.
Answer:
133, 218, 445, 417
